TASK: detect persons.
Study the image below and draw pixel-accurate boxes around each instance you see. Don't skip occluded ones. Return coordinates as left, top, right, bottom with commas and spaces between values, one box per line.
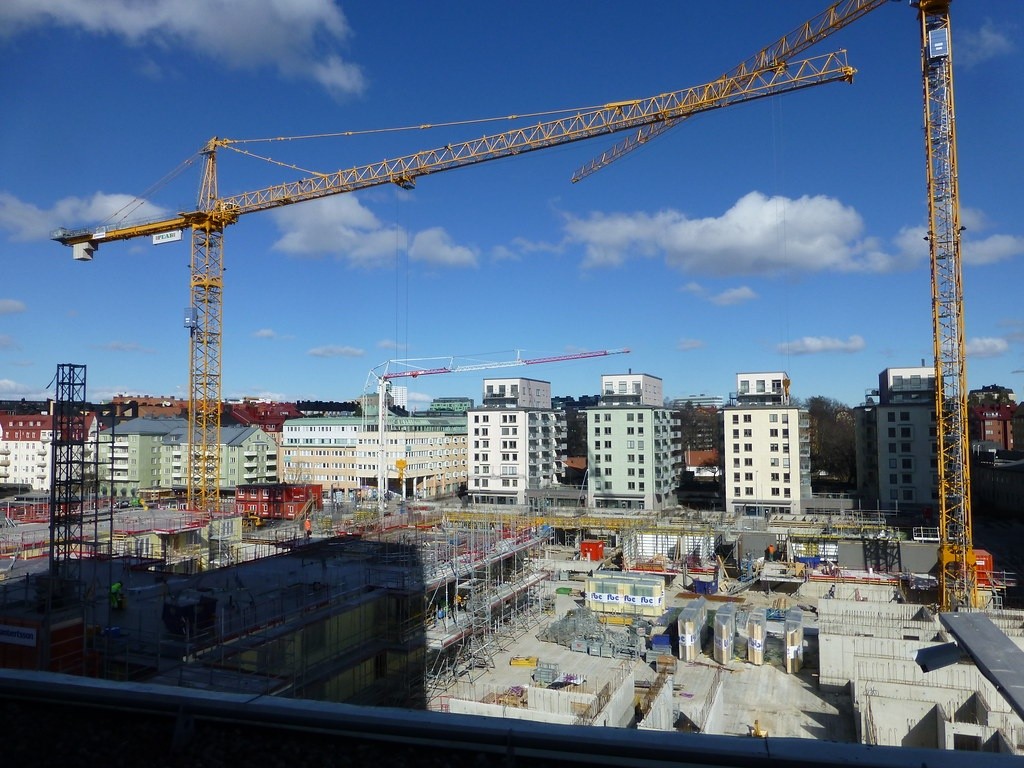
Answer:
458, 595, 461, 611
112, 581, 123, 608
305, 520, 312, 539
767, 544, 775, 562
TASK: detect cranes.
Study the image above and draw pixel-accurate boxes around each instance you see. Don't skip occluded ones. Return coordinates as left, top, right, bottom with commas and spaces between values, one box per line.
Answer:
365, 349, 632, 514
48, 47, 859, 511
569, 0, 980, 614
